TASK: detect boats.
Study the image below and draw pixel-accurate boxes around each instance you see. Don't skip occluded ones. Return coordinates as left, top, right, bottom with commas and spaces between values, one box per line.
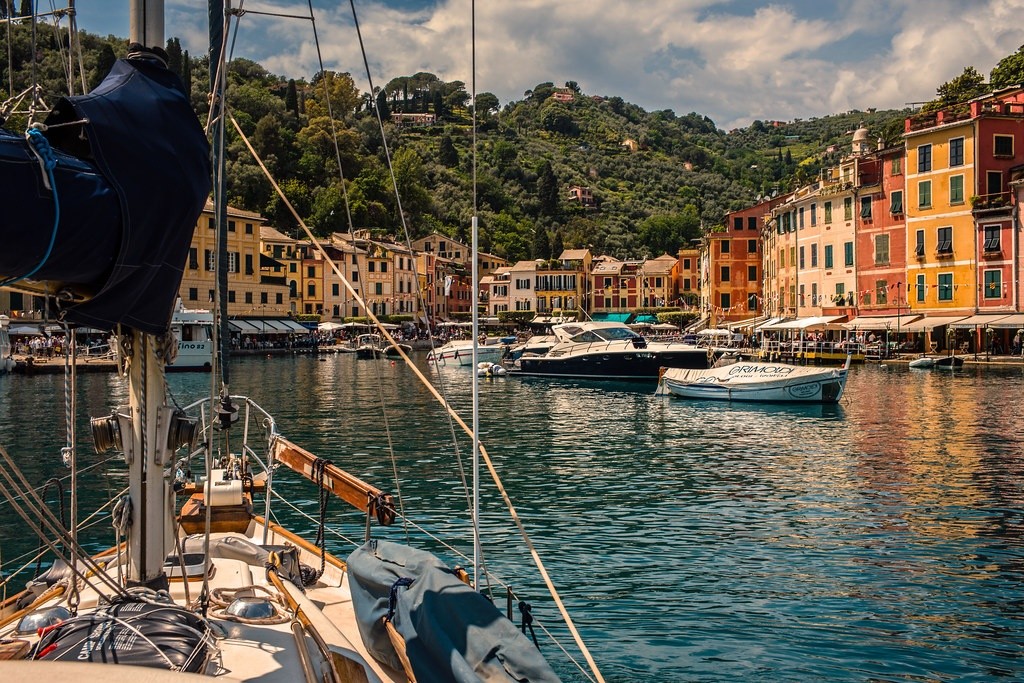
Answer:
162, 297, 215, 372
355, 334, 383, 358
909, 358, 935, 368
504, 321, 753, 378
662, 353, 852, 402
936, 356, 964, 371
382, 344, 412, 359
426, 339, 504, 366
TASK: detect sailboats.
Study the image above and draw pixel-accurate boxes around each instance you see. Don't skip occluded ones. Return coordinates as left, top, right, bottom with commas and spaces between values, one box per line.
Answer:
0, 0, 604, 683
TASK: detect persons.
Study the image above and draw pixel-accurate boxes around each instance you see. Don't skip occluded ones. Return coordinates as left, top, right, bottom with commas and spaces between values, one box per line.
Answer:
16, 335, 69, 356
87, 334, 106, 347
232, 328, 462, 351
770, 332, 879, 344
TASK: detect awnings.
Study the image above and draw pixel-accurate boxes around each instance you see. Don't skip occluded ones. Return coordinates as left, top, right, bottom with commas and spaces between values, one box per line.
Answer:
228, 320, 310, 334
6, 324, 42, 335
716, 315, 1024, 335
634, 315, 654, 322
260, 253, 285, 276
532, 317, 575, 324
589, 312, 631, 323
685, 318, 709, 333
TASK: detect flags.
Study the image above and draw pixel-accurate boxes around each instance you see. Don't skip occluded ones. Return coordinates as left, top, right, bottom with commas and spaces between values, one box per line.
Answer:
237, 271, 641, 315
754, 278, 1024, 307
642, 273, 753, 311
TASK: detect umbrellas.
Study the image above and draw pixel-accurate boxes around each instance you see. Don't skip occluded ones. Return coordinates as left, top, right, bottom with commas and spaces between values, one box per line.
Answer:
651, 323, 676, 332
437, 322, 473, 330
697, 328, 733, 340
372, 323, 398, 329
343, 322, 372, 326
320, 322, 346, 330
